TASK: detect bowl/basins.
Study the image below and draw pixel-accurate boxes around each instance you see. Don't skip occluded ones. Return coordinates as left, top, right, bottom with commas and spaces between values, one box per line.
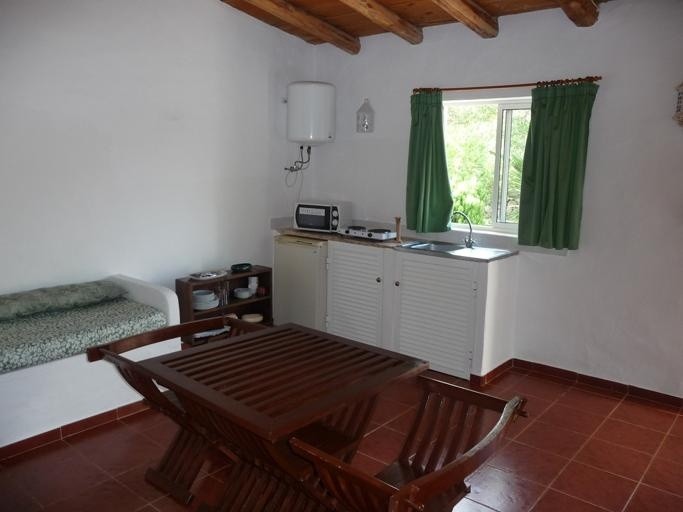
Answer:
230, 262, 252, 272
192, 289, 215, 302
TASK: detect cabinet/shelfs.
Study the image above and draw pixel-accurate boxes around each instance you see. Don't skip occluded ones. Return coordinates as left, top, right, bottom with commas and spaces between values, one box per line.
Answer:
172, 263, 273, 346
325, 238, 519, 380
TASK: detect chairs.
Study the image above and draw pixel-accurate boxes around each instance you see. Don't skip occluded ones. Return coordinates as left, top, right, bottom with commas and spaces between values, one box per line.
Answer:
84, 315, 529, 512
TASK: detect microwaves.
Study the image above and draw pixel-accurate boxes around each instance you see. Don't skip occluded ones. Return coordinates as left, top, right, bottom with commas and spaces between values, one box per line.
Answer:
293, 199, 352, 233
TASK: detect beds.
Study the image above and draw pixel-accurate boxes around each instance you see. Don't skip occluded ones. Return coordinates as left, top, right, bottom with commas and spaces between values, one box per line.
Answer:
0, 274, 180, 470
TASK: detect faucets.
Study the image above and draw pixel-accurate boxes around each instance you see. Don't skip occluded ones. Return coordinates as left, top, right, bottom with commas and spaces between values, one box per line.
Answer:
453, 210, 478, 247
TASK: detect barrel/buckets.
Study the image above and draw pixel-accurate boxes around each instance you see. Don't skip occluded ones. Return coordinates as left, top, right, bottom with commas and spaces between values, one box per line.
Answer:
357, 97, 374, 133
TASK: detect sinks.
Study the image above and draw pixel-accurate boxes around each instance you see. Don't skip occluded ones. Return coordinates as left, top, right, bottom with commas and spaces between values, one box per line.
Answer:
408, 240, 464, 253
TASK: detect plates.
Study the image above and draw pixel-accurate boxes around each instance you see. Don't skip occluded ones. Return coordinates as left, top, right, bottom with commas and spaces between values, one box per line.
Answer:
234, 287, 253, 299
241, 313, 264, 323
192, 295, 219, 311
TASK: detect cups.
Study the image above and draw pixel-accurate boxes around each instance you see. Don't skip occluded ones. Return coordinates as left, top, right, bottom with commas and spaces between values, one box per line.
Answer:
247, 277, 259, 296
